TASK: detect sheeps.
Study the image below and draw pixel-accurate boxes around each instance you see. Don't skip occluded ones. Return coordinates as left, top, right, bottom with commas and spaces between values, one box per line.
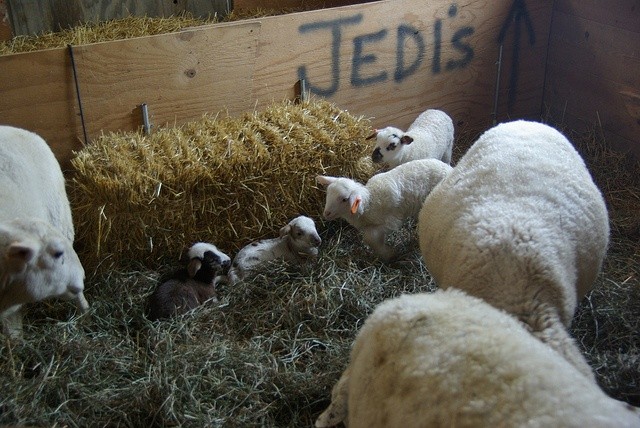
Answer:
0, 123, 91, 344
365, 107, 455, 169
229, 215, 322, 283
417, 120, 611, 388
312, 286, 640, 427
316, 158, 453, 264
145, 242, 231, 321
